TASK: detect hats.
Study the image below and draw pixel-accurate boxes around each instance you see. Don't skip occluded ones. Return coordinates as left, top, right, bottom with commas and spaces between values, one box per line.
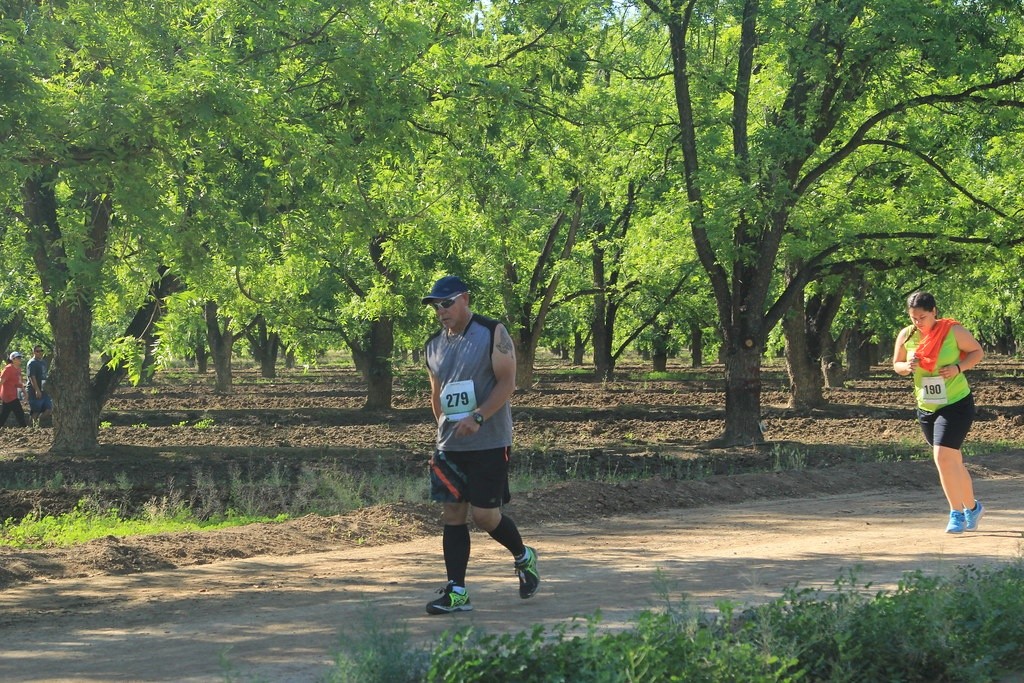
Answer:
9, 352, 21, 360
421, 276, 468, 304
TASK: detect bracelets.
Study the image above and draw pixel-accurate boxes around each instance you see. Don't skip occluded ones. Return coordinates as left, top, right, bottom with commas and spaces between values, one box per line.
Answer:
956, 364, 961, 373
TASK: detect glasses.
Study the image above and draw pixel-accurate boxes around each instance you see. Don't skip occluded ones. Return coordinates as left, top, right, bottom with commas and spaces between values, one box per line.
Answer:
432, 294, 463, 311
34, 350, 43, 353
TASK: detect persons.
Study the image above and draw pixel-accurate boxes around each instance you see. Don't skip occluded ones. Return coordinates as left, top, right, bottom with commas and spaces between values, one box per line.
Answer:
893, 293, 985, 535
0, 352, 26, 428
420, 275, 540, 614
25, 344, 52, 428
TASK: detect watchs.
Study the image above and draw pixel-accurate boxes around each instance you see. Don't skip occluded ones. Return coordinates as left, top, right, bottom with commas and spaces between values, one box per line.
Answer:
472, 413, 483, 426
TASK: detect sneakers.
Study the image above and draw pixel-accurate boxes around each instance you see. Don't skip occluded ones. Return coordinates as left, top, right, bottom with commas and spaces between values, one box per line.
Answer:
426, 583, 472, 615
946, 510, 965, 534
963, 500, 984, 532
515, 546, 540, 600
